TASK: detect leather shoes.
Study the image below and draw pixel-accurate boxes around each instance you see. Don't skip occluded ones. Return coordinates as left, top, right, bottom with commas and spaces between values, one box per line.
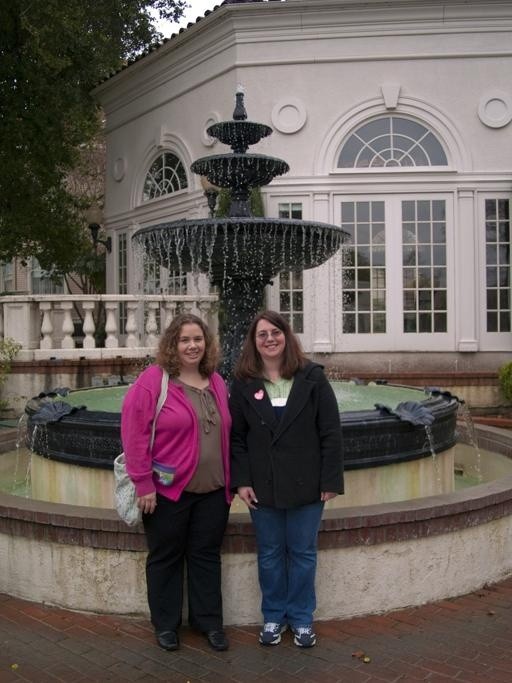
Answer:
205, 628, 228, 651
156, 630, 179, 650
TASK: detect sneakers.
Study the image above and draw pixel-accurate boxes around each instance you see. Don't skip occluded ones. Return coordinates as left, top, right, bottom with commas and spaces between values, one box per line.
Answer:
290, 626, 316, 647
258, 622, 289, 645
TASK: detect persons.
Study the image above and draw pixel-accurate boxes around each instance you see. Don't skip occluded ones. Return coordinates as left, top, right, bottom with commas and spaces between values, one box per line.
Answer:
228, 310, 345, 649
121, 314, 233, 652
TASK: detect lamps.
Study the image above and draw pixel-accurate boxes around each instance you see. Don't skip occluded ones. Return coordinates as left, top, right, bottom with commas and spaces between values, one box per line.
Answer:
88, 222, 111, 254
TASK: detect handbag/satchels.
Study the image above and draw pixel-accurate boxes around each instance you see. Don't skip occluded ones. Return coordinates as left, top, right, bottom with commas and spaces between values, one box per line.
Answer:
113, 452, 143, 528
